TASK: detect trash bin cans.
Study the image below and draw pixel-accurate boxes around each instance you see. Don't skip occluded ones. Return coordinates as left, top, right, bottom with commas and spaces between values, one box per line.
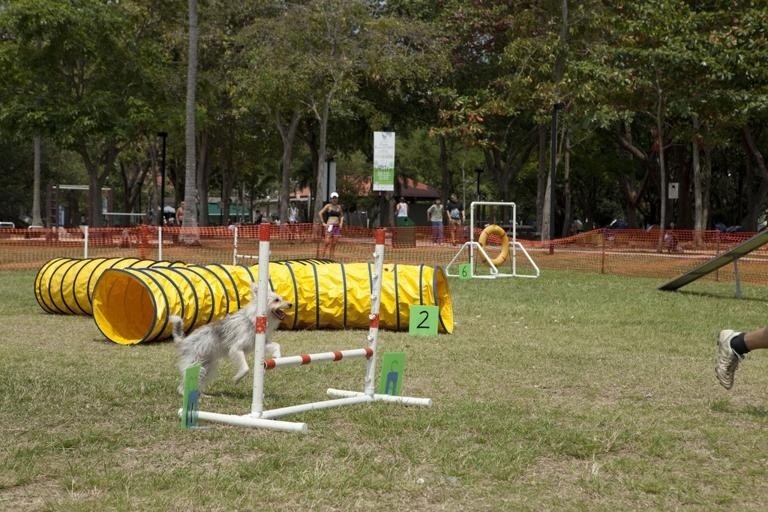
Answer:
392, 217, 417, 249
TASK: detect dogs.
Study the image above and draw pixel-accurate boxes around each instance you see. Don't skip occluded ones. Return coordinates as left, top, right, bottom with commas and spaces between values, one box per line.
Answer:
165, 278, 294, 403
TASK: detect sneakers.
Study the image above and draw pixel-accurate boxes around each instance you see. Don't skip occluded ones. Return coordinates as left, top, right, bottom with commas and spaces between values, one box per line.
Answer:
714, 330, 745, 389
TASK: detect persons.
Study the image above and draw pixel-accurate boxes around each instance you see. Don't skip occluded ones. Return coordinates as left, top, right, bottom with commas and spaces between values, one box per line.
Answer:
273, 217, 280, 225
573, 217, 584, 234
716, 326, 768, 389
447, 193, 465, 247
288, 201, 299, 240
427, 198, 445, 246
254, 210, 261, 224
395, 196, 408, 217
80, 213, 87, 224
175, 202, 184, 227
319, 191, 344, 260
162, 213, 176, 227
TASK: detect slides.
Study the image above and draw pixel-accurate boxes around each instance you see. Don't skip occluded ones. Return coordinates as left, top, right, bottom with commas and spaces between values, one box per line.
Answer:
658, 231, 767, 290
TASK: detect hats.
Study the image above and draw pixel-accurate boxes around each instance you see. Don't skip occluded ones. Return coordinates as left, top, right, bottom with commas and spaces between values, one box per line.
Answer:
330, 192, 338, 198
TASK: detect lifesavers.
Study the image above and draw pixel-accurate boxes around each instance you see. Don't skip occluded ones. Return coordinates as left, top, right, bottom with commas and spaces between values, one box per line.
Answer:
478, 224, 509, 265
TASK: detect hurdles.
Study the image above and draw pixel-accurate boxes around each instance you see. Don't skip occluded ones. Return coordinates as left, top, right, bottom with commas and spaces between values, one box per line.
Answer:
177, 224, 432, 433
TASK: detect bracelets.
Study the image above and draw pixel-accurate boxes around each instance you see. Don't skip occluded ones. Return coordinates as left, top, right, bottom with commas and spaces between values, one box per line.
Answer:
321, 220, 325, 223
